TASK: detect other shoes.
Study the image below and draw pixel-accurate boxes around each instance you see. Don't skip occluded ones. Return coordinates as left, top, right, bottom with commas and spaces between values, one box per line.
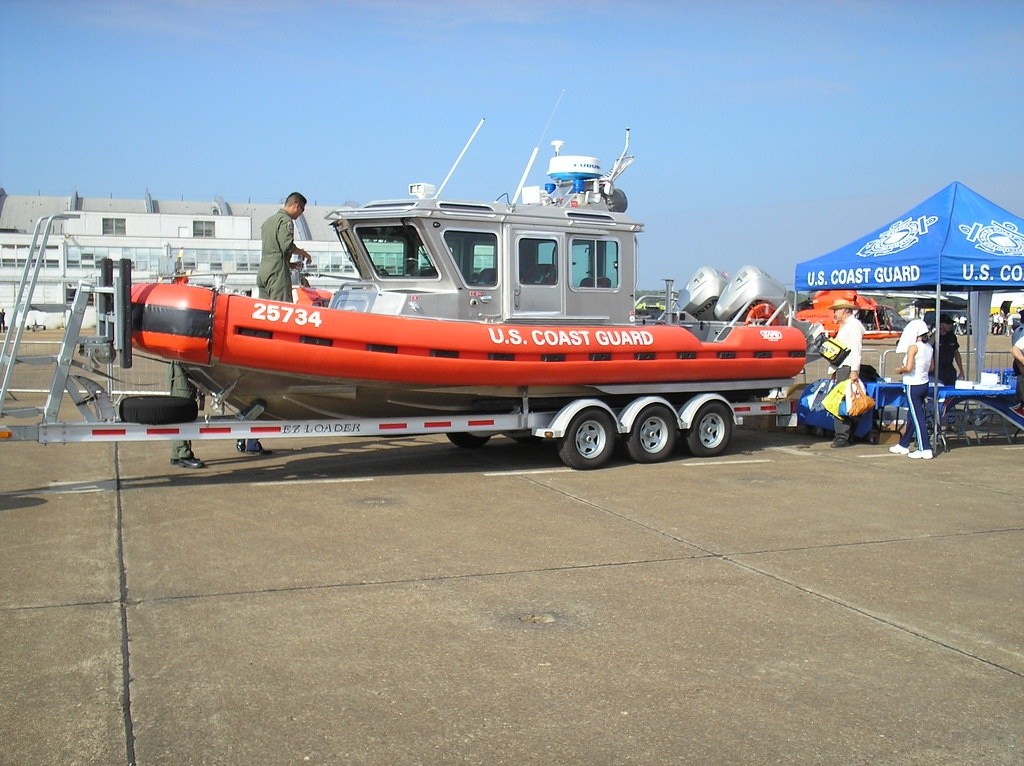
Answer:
237, 440, 245, 452
247, 449, 273, 455
831, 440, 848, 448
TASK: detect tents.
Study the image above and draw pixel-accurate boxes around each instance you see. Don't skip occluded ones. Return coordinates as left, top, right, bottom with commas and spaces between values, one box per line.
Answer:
793, 181, 1024, 454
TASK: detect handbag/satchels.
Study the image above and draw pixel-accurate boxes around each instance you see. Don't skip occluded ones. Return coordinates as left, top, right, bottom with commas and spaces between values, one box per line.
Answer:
822, 378, 875, 421
818, 336, 851, 366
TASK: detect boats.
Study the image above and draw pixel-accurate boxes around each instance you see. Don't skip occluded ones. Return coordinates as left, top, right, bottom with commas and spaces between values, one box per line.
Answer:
124, 88, 811, 388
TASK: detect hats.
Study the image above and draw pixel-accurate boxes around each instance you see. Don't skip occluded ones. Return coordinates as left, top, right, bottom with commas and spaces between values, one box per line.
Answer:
940, 314, 955, 324
827, 300, 856, 310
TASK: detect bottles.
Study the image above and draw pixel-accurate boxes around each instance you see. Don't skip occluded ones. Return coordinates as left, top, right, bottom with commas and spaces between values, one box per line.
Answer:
987, 368, 1015, 384
297, 248, 304, 270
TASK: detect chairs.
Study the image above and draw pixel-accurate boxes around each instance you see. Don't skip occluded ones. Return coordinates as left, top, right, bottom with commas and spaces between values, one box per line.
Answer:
520, 263, 557, 285
925, 397, 1013, 445
578, 276, 612, 288
475, 268, 498, 285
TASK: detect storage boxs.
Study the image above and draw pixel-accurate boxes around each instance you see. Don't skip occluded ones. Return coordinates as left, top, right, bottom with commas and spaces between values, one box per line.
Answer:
740, 411, 835, 439
873, 418, 908, 444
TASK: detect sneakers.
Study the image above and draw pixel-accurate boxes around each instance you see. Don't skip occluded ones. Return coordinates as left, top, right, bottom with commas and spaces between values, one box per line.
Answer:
908, 450, 933, 459
889, 444, 910, 455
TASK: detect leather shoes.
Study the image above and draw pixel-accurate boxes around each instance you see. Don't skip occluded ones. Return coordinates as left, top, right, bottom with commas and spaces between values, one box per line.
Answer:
179, 457, 204, 469
170, 458, 179, 465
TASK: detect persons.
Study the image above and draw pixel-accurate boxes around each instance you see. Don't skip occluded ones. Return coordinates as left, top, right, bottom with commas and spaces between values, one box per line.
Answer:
827, 300, 866, 448
927, 313, 965, 433
989, 310, 1014, 337
236, 439, 273, 455
170, 360, 203, 468
0, 309, 5, 333
889, 319, 935, 459
256, 192, 312, 304
1012, 336, 1024, 367
960, 313, 968, 334
1012, 310, 1024, 403
953, 314, 963, 336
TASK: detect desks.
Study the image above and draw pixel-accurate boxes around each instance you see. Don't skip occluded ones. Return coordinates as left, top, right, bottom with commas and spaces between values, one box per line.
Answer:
862, 382, 910, 445
926, 386, 1021, 453
